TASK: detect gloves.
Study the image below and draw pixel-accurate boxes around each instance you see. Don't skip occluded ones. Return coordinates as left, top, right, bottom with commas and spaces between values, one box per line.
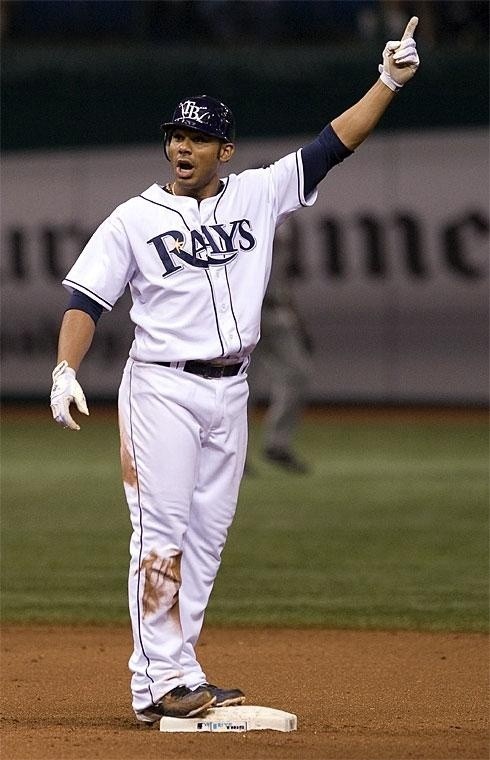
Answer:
378, 16, 420, 95
50, 360, 90, 431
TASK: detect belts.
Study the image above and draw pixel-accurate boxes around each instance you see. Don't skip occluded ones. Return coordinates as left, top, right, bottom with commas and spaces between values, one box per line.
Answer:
156, 358, 247, 378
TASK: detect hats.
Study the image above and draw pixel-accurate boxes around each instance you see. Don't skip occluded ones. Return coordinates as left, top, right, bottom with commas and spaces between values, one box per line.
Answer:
160, 94, 237, 143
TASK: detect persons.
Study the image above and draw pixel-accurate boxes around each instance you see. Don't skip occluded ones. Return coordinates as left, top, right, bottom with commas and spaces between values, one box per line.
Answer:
241, 239, 312, 485
43, 11, 422, 722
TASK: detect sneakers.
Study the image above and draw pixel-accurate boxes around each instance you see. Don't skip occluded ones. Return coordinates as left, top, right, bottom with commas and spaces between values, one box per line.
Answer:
135, 683, 246, 722
